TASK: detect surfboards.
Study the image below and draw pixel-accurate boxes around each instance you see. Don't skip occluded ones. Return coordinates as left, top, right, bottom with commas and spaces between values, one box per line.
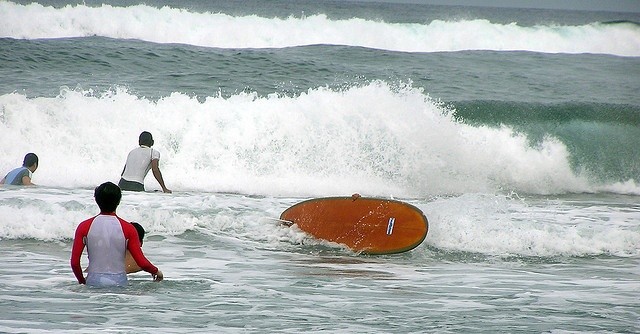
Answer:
280, 196, 429, 254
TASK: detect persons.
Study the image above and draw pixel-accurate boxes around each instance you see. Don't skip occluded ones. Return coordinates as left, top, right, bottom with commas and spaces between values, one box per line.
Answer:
125, 221, 145, 274
71, 181, 163, 288
118, 131, 172, 193
0, 153, 39, 186
